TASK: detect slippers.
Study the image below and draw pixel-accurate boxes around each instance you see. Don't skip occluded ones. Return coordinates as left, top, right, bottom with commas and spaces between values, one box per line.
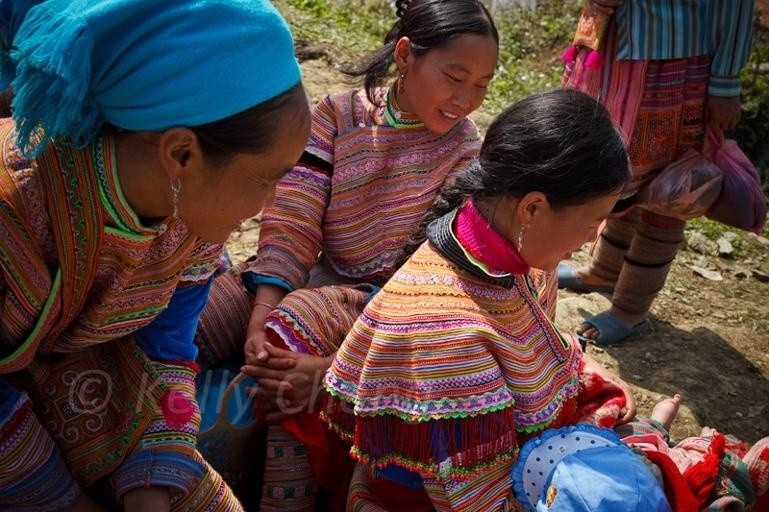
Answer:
557, 264, 614, 292
574, 310, 651, 347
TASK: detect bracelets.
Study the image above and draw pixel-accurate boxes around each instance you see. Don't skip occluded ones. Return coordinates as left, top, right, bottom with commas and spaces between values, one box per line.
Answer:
252, 301, 273, 310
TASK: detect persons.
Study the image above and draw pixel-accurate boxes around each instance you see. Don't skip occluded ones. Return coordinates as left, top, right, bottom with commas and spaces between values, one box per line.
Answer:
192, 0, 504, 510
1, 0, 313, 512
320, 90, 769, 512
528, 391, 755, 511
556, 2, 757, 347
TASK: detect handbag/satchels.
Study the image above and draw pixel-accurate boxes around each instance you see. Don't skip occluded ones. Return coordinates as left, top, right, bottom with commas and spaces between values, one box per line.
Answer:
572, 2, 614, 51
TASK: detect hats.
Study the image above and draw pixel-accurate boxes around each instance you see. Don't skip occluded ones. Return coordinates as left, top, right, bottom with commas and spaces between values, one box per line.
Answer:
513, 423, 671, 512
